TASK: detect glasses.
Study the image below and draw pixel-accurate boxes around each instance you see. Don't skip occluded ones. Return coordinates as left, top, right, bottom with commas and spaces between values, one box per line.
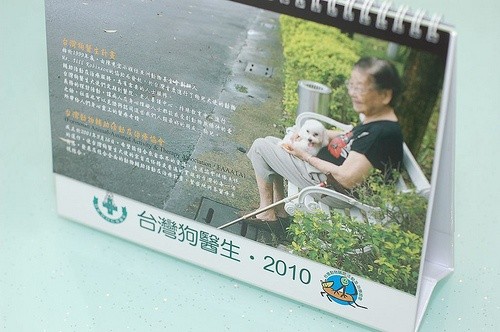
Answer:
345, 80, 381, 95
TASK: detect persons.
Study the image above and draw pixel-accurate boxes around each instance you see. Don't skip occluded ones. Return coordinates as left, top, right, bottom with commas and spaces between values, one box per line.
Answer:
248, 56, 402, 222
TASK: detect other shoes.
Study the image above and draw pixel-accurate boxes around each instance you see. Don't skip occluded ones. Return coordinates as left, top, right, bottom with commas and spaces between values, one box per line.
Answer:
237, 211, 279, 232
275, 216, 290, 232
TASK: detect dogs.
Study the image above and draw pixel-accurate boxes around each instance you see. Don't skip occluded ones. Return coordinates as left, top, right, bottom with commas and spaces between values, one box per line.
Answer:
283, 119, 337, 157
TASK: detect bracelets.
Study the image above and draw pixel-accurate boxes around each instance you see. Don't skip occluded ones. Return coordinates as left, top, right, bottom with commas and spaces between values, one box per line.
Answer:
307, 155, 312, 162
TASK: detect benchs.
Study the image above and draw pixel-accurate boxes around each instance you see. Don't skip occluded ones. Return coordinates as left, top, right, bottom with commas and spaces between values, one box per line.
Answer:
216, 112, 431, 256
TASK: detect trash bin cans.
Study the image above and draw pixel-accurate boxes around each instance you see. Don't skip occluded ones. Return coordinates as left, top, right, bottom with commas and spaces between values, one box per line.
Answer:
296, 79, 332, 128
